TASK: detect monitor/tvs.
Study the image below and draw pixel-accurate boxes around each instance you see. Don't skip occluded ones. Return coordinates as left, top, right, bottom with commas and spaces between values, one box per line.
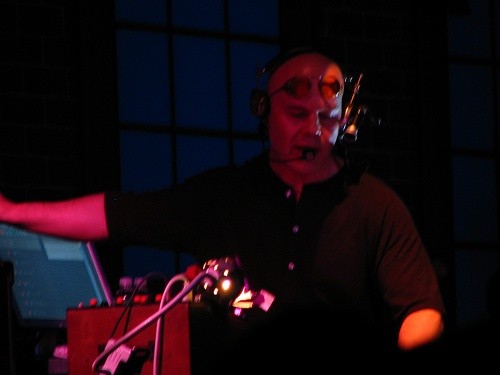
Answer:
0, 223, 114, 329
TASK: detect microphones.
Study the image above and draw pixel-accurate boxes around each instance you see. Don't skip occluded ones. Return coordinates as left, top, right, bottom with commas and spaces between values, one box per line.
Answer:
144, 271, 166, 304
266, 146, 316, 166
341, 104, 367, 141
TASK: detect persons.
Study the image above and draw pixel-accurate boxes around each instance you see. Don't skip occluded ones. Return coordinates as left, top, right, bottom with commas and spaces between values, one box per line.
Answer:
0, 44, 447, 374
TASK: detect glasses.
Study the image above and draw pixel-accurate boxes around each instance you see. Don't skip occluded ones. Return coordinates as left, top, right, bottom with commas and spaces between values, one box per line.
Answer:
263, 74, 342, 100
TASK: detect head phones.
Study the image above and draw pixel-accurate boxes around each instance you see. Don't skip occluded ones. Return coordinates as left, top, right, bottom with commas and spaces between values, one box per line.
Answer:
248, 46, 354, 122
